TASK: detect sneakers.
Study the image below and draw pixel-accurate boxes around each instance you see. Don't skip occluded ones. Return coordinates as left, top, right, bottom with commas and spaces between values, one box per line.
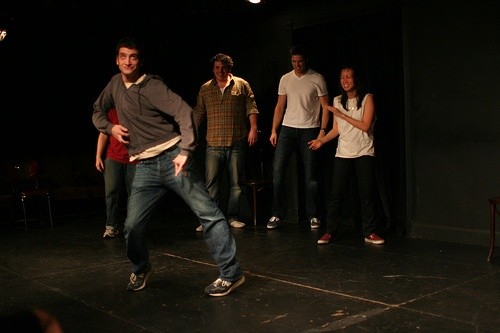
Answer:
317, 233, 332, 244
364, 233, 385, 244
204, 273, 245, 297
310, 217, 321, 229
128, 266, 154, 290
102, 225, 120, 238
229, 219, 245, 228
196, 225, 203, 232
266, 215, 280, 229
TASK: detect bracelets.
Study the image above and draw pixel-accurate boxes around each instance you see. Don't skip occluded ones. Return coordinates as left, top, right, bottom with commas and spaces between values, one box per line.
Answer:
320, 128, 326, 130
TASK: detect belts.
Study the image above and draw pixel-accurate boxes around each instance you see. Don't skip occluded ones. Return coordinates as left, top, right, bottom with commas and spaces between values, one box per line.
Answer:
137, 145, 177, 160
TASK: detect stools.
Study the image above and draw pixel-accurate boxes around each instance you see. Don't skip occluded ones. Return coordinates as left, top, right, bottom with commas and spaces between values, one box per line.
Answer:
486, 196, 500, 262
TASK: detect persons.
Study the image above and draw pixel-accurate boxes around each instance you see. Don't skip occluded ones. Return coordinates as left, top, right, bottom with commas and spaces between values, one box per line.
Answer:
266, 47, 330, 231
91, 38, 245, 298
194, 53, 260, 233
308, 64, 385, 246
95, 103, 136, 239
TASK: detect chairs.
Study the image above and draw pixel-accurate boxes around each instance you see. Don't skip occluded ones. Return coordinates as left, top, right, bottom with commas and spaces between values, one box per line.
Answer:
7, 160, 55, 233
